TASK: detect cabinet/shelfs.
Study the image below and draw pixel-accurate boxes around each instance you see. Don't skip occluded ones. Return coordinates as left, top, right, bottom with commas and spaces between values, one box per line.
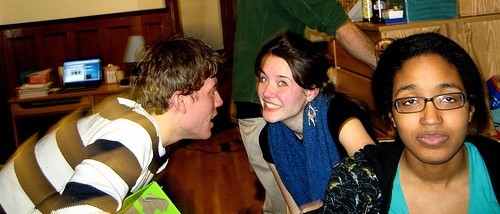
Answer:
6, 83, 129, 148
322, 0, 500, 142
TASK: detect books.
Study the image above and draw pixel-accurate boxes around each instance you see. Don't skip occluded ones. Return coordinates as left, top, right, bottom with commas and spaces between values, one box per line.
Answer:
18, 81, 54, 99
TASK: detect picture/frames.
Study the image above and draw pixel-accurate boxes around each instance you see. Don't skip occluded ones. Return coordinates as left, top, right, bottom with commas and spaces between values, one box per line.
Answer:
102, 66, 119, 84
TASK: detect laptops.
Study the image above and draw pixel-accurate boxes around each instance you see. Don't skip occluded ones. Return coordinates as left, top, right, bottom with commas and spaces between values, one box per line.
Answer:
55, 59, 104, 94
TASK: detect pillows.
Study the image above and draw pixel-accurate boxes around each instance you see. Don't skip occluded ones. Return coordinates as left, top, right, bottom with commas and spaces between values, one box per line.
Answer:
238, 116, 286, 214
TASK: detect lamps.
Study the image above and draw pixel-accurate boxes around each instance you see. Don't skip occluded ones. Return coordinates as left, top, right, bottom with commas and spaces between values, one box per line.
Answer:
120, 35, 146, 87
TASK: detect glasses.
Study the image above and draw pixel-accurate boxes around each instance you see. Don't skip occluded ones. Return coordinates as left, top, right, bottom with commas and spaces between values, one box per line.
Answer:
393, 92, 472, 116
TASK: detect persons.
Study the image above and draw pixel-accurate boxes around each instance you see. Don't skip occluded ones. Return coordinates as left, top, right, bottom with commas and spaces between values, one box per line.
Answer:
232, 0, 378, 214
255, 30, 379, 214
323, 32, 500, 214
0, 36, 224, 214
